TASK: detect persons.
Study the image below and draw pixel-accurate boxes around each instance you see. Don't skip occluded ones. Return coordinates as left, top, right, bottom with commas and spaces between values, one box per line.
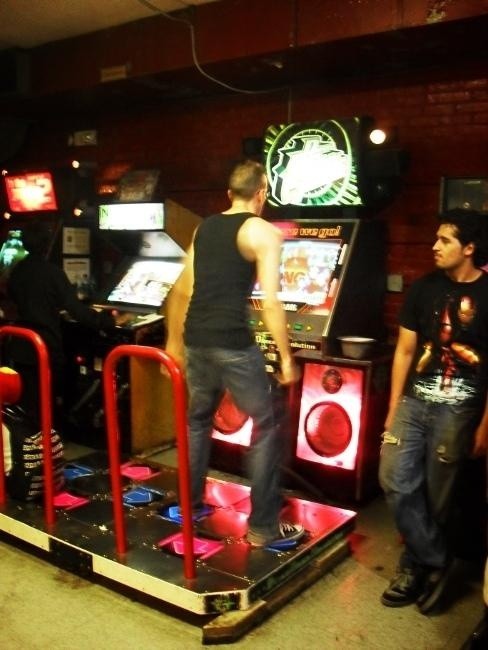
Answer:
158, 160, 304, 547
377, 204, 486, 613
0, 220, 123, 433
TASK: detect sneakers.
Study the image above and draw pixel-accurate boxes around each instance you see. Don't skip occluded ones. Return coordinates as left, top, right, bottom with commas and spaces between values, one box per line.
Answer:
70, 381, 100, 410
415, 553, 458, 612
380, 564, 424, 608
192, 503, 214, 520
249, 523, 305, 547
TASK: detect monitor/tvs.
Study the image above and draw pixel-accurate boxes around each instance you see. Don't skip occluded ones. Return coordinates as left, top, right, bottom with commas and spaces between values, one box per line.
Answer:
94, 256, 186, 314
1, 239, 30, 280
244, 216, 386, 350
